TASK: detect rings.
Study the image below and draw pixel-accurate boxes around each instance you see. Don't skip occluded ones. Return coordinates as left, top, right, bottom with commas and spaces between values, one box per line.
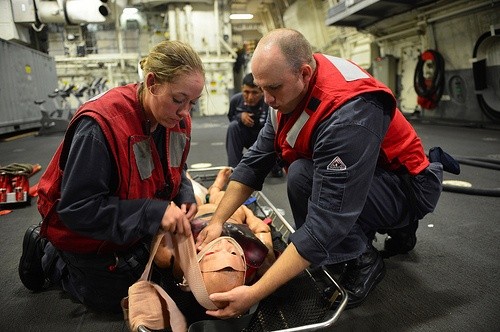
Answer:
238, 315, 241, 318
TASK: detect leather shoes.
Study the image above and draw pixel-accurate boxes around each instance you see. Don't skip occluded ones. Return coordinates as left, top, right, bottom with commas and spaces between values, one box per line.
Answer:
396, 215, 418, 251
320, 252, 385, 308
19, 225, 47, 291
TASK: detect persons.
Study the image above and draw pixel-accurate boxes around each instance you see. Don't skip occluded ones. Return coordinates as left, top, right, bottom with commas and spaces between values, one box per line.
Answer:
151, 167, 275, 310
227, 74, 284, 178
18, 41, 205, 309
194, 28, 441, 319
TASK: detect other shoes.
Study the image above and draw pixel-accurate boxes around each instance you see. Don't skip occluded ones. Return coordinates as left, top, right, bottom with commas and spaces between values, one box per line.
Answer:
271, 168, 283, 177
